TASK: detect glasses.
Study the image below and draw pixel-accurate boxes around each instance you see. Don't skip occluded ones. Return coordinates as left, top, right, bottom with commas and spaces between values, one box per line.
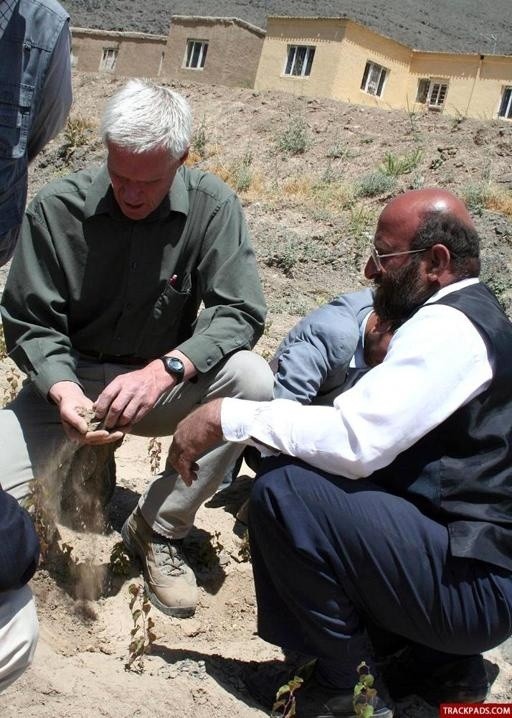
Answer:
369, 244, 430, 273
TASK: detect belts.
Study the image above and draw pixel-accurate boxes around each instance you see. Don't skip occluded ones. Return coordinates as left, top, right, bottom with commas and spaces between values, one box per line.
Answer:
76, 348, 148, 366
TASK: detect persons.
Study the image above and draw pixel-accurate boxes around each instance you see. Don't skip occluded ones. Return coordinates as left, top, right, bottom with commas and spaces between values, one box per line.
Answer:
1, 486, 41, 691
1, 77, 278, 623
168, 186, 511, 717
242, 284, 399, 474
1, 0, 76, 272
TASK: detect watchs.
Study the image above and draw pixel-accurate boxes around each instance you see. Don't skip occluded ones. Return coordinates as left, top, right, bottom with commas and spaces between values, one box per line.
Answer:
159, 354, 186, 386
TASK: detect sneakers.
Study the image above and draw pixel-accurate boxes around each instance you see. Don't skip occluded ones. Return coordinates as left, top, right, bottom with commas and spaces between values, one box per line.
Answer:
240, 659, 397, 718
82, 434, 126, 509
120, 503, 200, 619
384, 653, 492, 708
